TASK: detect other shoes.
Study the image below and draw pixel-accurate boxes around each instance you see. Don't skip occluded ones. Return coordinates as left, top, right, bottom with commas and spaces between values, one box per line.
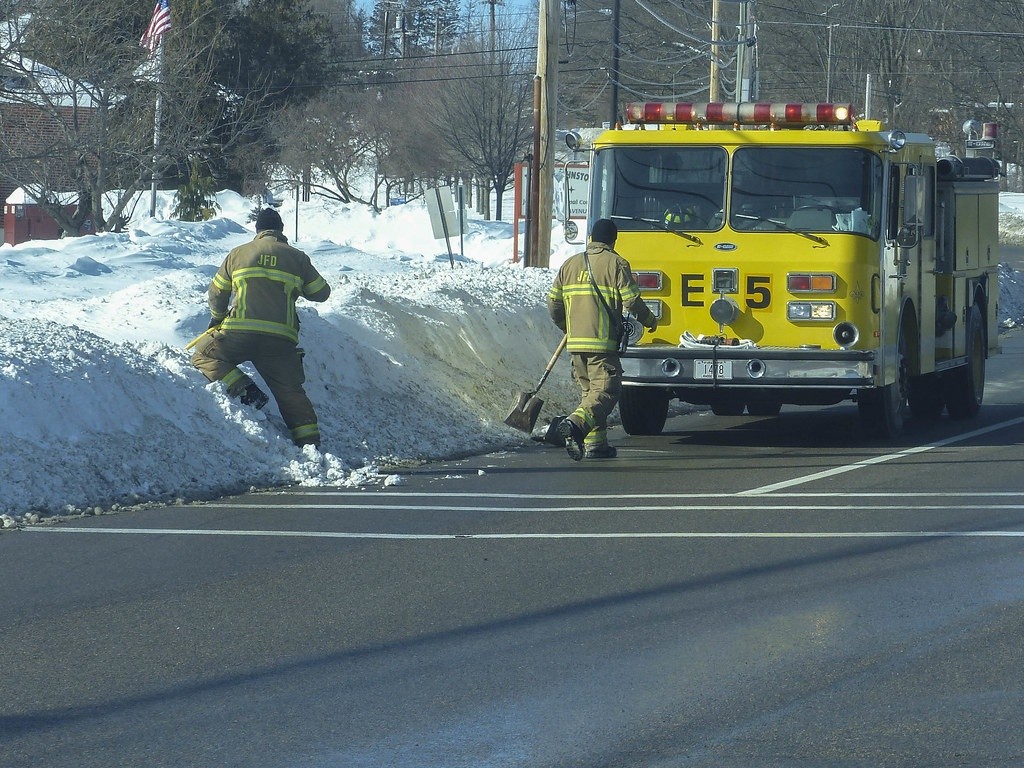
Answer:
238, 383, 269, 411
558, 419, 585, 461
584, 443, 617, 459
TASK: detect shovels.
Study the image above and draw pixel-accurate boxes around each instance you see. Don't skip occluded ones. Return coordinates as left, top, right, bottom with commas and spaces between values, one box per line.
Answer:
502, 332, 569, 434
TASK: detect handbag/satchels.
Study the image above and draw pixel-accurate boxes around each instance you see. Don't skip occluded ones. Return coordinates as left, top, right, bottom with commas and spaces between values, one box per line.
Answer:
615, 327, 631, 357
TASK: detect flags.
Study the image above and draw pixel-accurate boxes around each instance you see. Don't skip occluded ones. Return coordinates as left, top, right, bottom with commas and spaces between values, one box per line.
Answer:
140, 0, 172, 51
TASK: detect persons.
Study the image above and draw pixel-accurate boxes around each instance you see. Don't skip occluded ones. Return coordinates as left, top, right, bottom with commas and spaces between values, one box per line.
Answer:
548, 220, 657, 462
191, 209, 330, 450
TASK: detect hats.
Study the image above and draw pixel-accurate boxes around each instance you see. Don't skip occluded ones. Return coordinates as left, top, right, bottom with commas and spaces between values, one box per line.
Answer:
255, 207, 284, 234
591, 218, 618, 246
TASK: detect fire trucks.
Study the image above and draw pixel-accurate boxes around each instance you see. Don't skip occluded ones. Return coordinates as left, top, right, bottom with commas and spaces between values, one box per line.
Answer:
562, 98, 1007, 440
